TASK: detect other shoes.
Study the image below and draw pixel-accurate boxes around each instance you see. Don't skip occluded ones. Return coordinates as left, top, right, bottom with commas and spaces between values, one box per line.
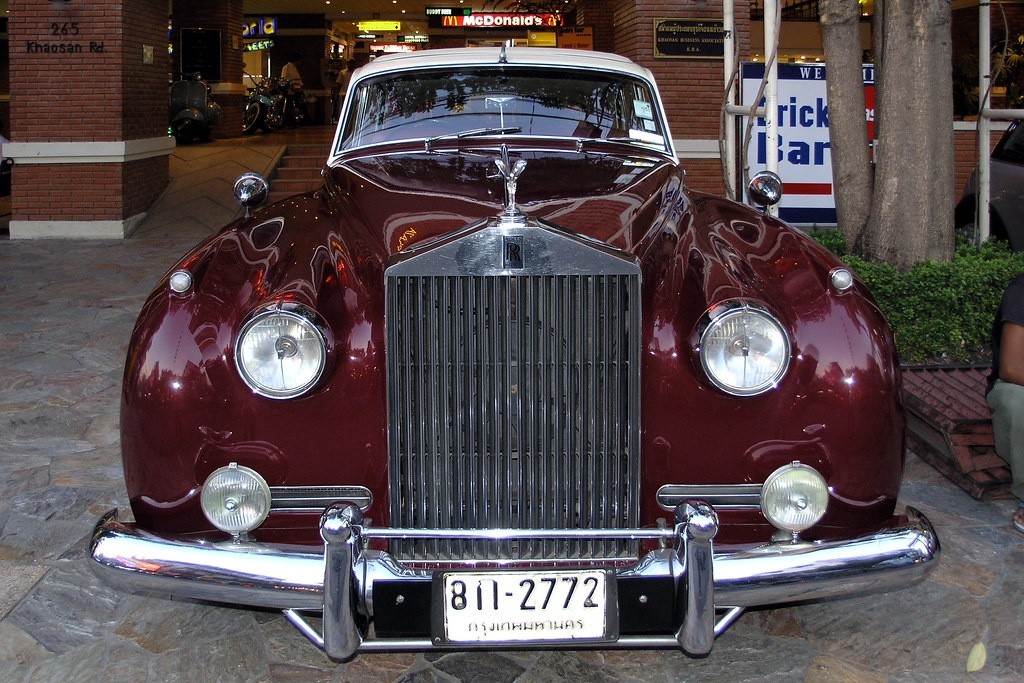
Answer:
1013, 503, 1024, 533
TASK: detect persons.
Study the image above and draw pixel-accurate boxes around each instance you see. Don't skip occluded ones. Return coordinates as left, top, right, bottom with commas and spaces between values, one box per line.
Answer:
984, 272, 1024, 533
335, 57, 358, 110
281, 53, 316, 127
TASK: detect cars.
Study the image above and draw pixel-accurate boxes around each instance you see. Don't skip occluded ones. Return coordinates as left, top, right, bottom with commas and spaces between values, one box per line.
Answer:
955, 119, 1024, 252
86, 47, 943, 661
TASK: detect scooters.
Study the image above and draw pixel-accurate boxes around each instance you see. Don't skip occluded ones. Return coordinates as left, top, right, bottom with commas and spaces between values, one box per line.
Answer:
243, 71, 306, 134
169, 71, 222, 145
